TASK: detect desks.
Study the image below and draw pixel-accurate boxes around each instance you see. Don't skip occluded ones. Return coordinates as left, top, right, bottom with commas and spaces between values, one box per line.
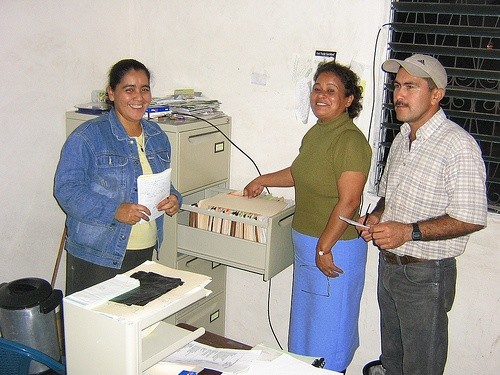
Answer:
176, 323, 253, 351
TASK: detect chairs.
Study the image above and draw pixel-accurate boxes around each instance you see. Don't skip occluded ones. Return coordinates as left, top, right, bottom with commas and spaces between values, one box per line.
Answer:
0, 338, 64, 375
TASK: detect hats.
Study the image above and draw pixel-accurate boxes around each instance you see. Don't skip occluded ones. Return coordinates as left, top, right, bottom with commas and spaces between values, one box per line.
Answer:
382, 53, 448, 90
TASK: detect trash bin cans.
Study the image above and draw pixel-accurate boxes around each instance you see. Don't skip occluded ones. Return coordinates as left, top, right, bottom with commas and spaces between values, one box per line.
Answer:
0, 275, 64, 375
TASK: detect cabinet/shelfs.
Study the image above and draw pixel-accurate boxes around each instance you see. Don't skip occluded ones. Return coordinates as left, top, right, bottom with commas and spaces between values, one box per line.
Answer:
62, 260, 212, 374
65, 110, 295, 337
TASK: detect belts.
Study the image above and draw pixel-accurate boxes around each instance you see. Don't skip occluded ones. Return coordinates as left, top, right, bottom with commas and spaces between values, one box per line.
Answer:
379, 248, 428, 265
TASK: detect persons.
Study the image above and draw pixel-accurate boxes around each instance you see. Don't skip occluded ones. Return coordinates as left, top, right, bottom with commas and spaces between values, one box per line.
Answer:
243, 60, 372, 375
54, 59, 183, 297
354, 54, 488, 375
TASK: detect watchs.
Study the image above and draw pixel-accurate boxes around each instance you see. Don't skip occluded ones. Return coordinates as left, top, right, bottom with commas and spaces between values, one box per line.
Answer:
412, 223, 422, 241
316, 247, 331, 256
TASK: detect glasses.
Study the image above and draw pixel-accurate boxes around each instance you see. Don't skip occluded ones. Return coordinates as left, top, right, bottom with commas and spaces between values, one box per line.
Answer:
300, 265, 330, 297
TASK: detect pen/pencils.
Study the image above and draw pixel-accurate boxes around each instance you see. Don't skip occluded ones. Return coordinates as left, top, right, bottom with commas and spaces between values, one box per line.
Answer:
357, 202, 372, 240
